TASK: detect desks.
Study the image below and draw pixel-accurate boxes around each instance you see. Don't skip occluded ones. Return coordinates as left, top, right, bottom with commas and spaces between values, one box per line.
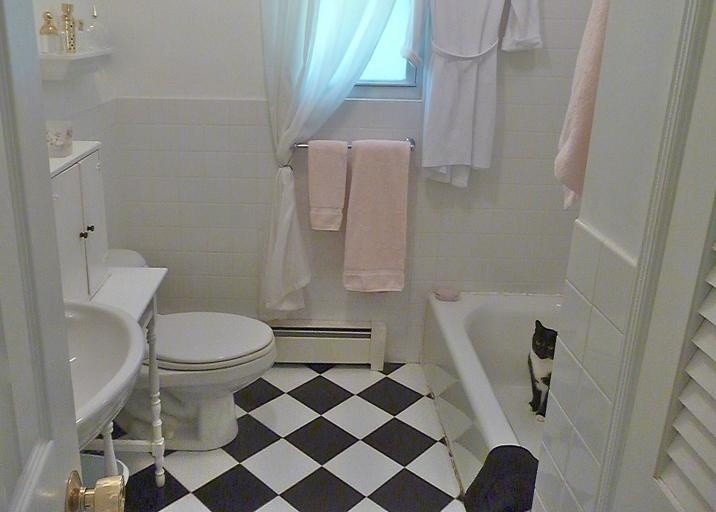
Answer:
90, 268, 169, 488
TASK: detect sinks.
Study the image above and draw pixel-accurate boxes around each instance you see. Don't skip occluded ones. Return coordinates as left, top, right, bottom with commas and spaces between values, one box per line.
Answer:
63, 298, 146, 451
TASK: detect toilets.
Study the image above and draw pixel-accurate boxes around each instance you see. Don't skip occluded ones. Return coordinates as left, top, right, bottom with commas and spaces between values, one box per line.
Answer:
108, 247, 278, 451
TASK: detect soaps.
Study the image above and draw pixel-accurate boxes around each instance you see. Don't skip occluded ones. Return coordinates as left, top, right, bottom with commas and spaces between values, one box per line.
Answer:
437, 287, 458, 299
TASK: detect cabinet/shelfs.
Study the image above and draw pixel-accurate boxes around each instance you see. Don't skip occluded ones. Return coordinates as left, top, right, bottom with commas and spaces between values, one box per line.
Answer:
51, 143, 113, 302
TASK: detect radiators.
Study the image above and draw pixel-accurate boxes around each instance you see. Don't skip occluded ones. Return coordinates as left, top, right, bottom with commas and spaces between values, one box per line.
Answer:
265, 320, 386, 371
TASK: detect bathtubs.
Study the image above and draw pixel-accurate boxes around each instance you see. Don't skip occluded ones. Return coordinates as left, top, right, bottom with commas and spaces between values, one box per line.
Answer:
421, 293, 564, 494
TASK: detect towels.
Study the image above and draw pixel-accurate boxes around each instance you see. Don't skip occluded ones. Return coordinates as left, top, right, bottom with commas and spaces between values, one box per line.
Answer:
342, 140, 411, 294
307, 140, 347, 232
554, 0, 631, 214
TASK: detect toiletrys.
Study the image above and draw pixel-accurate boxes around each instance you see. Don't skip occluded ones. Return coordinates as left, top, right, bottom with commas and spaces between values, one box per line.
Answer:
36, 4, 102, 60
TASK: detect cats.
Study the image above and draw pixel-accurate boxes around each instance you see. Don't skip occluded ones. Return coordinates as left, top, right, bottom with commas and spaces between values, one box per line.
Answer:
528, 319, 558, 422
